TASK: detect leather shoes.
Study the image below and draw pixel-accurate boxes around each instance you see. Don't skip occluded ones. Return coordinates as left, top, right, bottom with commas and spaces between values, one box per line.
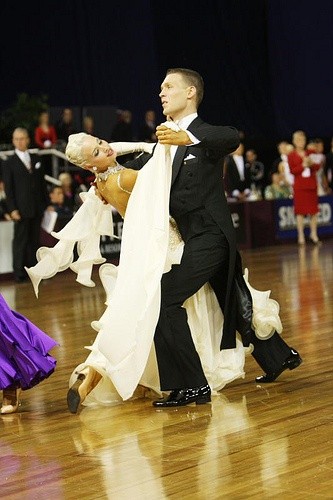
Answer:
255, 348, 302, 383
153, 383, 212, 407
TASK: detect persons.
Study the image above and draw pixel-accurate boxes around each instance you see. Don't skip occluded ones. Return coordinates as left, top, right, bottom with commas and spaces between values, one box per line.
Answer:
0, 108, 159, 281
225, 132, 333, 246
27, 68, 304, 413
0, 294, 60, 414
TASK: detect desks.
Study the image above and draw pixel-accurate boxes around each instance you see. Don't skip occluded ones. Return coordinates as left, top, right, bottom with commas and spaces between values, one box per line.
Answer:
228, 195, 333, 252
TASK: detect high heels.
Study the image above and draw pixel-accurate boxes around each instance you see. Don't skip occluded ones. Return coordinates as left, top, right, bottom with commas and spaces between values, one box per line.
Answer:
67, 365, 97, 413
0, 381, 21, 413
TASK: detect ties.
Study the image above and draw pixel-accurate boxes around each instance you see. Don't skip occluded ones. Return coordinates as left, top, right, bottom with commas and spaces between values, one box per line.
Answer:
22, 154, 31, 171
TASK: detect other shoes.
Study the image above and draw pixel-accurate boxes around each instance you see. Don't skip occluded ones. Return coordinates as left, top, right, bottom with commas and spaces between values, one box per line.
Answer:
15, 276, 28, 281
